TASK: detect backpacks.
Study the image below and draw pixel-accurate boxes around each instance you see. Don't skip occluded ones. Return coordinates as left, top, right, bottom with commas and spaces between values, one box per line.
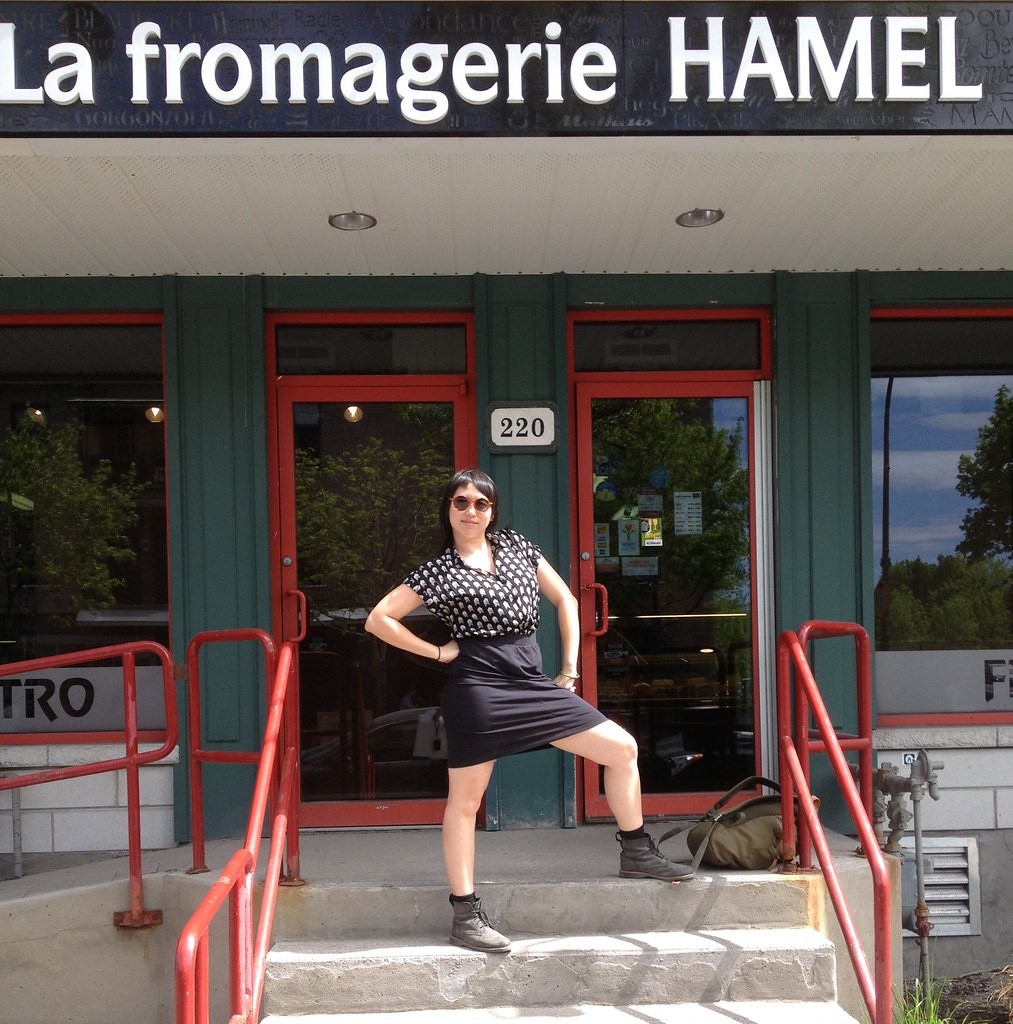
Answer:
656, 774, 820, 875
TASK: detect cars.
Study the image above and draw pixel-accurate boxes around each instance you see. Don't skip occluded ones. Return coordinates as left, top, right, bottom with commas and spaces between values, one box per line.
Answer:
328, 707, 452, 783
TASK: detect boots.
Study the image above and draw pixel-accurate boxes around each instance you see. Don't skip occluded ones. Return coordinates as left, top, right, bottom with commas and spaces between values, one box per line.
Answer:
619, 833, 696, 880
450, 898, 511, 953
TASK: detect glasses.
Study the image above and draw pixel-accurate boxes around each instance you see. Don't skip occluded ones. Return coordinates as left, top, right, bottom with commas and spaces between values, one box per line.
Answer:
446, 496, 493, 513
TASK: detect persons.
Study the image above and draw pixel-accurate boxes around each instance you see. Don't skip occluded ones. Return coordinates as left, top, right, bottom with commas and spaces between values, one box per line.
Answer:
364, 470, 697, 955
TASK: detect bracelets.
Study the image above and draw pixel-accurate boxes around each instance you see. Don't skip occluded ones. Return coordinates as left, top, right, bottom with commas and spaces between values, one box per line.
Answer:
560, 670, 581, 680
435, 646, 441, 661
